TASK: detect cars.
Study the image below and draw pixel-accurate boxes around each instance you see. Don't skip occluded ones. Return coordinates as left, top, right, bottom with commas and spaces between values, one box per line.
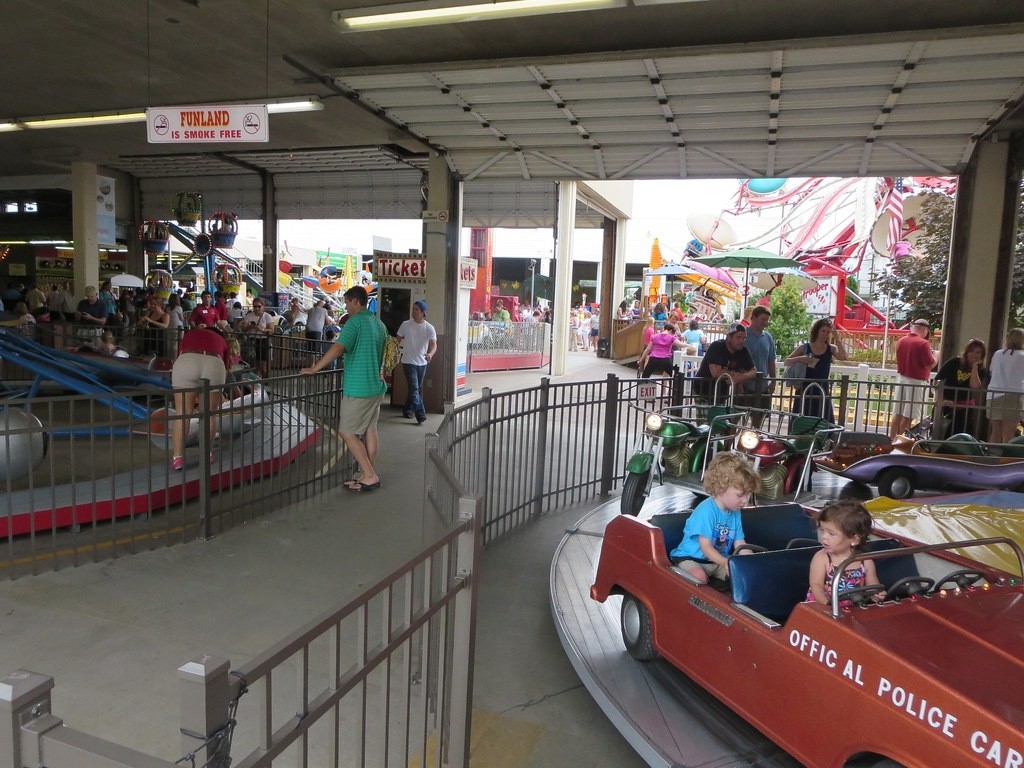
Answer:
588, 498, 1023, 768
813, 427, 1024, 502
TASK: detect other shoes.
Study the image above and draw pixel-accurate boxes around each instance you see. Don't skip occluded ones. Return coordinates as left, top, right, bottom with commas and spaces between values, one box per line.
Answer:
403, 410, 414, 419
418, 415, 426, 423
583, 348, 588, 351
593, 350, 598, 352
580, 347, 586, 349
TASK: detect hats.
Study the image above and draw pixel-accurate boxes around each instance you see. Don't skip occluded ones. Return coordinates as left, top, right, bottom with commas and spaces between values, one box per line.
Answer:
416, 300, 427, 319
727, 322, 745, 335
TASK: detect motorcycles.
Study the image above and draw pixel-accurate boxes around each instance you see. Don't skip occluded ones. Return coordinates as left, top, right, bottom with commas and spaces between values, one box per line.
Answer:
620, 372, 845, 520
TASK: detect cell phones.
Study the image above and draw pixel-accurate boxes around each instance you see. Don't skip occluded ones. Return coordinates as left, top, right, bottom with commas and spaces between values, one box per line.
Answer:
250, 321, 256, 325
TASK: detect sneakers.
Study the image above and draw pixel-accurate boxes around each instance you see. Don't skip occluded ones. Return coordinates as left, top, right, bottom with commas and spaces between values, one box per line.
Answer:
211, 454, 215, 466
171, 456, 183, 470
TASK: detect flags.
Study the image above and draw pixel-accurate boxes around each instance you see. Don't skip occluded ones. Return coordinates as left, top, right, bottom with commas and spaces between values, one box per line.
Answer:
882, 176, 903, 251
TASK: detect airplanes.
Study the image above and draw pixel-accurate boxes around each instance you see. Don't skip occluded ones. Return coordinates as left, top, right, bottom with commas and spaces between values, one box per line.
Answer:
126, 376, 270, 452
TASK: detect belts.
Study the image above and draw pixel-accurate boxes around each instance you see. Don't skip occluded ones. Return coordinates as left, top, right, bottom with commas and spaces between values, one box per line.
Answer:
181, 349, 217, 357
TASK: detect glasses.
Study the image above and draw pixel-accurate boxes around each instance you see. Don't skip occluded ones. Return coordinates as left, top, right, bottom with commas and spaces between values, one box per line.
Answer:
968, 338, 984, 344
254, 305, 263, 308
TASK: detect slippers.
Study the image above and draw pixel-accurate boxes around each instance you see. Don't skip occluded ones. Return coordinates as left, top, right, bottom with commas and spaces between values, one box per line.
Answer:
344, 477, 381, 491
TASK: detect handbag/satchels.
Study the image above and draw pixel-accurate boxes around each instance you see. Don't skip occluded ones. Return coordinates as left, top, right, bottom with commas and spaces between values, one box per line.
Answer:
781, 343, 808, 388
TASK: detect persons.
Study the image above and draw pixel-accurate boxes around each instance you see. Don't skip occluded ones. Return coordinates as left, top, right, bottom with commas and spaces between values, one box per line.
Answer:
691, 323, 757, 429
929, 339, 986, 441
637, 324, 689, 396
740, 306, 755, 327
890, 319, 939, 438
680, 319, 706, 381
670, 451, 761, 584
226, 338, 241, 365
396, 301, 437, 423
986, 328, 1024, 444
805, 500, 887, 608
660, 311, 681, 356
301, 286, 388, 492
784, 318, 847, 441
305, 299, 330, 369
642, 317, 655, 369
326, 330, 335, 342
468, 289, 734, 352
171, 326, 229, 470
0, 202, 305, 354
723, 305, 776, 450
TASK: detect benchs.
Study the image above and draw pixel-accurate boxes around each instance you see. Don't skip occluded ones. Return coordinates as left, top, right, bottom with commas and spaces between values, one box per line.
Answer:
650, 503, 815, 591
728, 536, 920, 623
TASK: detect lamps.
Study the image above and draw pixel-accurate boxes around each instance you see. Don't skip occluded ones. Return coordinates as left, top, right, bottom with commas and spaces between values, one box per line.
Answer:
0, 94, 326, 133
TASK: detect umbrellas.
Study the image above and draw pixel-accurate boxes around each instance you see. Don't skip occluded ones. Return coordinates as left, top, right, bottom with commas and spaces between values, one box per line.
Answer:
687, 260, 739, 287
688, 246, 807, 308
646, 260, 697, 303
